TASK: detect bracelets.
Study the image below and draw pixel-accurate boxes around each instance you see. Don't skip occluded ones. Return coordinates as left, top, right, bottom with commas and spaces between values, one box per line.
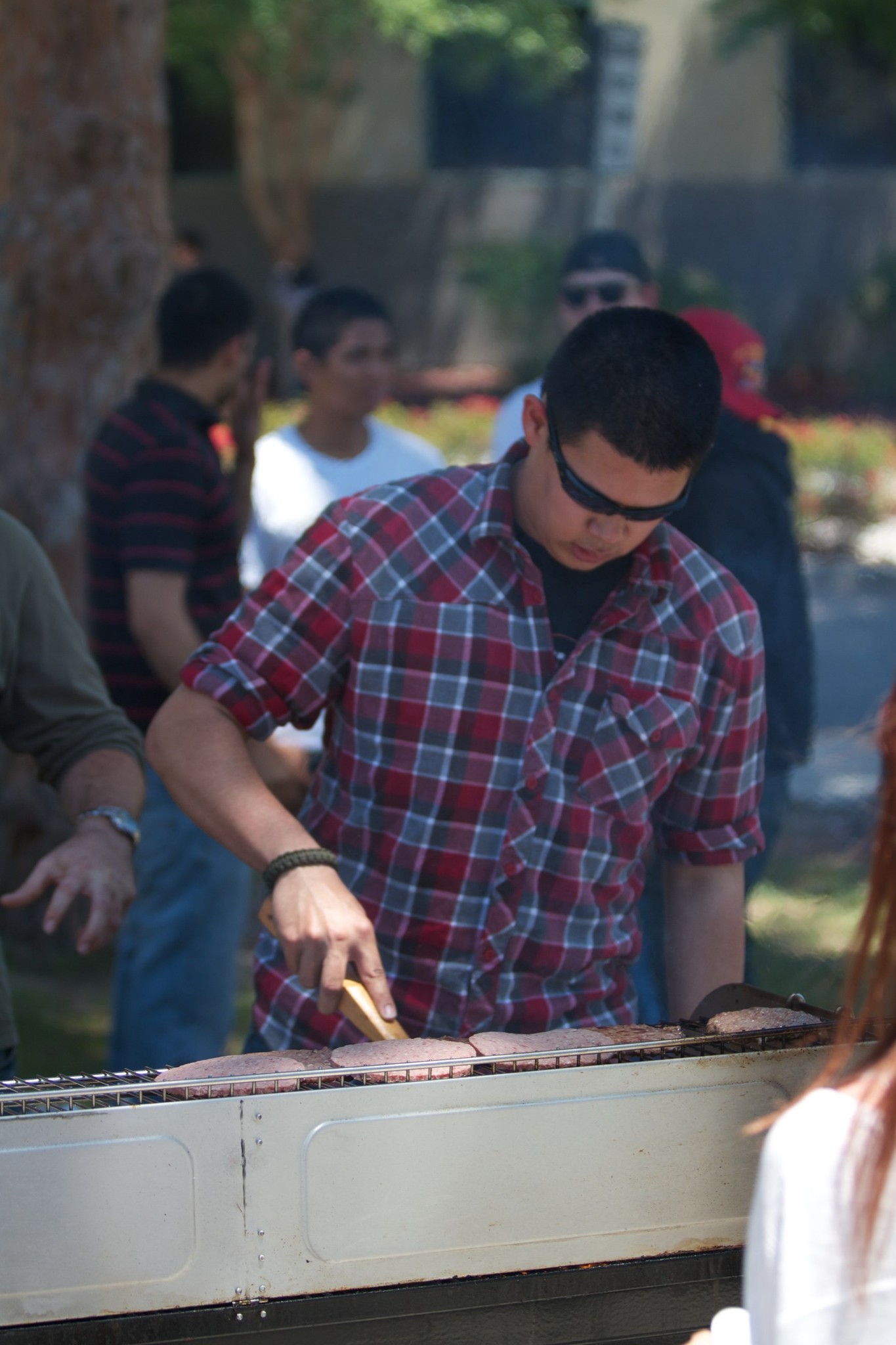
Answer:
263, 848, 339, 891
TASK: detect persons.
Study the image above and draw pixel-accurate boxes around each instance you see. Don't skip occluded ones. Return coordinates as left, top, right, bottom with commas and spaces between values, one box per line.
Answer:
141, 305, 768, 1054
0, 507, 145, 1082
76, 228, 818, 1087
741, 673, 896, 1345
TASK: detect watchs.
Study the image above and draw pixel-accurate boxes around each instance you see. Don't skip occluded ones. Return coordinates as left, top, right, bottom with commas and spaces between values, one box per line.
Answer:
73, 803, 143, 852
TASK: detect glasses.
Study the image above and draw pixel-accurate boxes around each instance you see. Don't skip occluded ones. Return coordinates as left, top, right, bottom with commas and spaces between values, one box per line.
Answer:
545, 408, 691, 520
560, 281, 631, 309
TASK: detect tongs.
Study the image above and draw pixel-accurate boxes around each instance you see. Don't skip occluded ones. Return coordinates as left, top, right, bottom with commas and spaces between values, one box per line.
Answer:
258, 896, 411, 1043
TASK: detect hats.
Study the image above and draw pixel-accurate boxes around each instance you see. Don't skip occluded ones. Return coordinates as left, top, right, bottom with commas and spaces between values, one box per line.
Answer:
678, 307, 785, 425
564, 232, 651, 281
